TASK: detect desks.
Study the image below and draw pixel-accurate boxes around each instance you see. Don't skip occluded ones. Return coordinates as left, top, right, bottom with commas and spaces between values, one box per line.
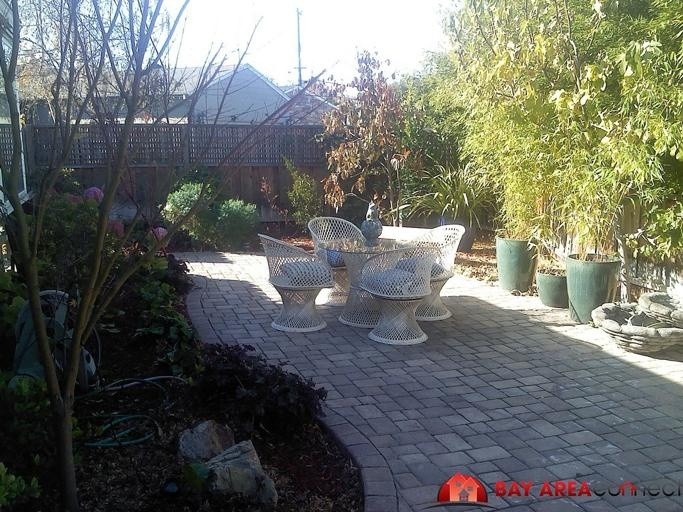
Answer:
318, 238, 418, 329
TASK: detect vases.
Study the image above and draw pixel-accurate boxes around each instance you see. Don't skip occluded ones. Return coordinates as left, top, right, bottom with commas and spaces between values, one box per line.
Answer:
361, 200, 382, 247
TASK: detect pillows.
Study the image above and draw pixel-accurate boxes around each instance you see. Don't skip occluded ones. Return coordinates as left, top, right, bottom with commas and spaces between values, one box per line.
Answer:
363, 268, 427, 296
327, 247, 363, 267
396, 257, 444, 278
279, 262, 333, 285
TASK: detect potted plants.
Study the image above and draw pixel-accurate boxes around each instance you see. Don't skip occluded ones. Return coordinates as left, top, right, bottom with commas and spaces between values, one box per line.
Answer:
495, 131, 545, 292
566, 97, 646, 326
404, 160, 506, 253
527, 138, 569, 308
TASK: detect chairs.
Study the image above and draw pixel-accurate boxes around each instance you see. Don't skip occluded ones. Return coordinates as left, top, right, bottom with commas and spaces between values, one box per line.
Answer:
308, 217, 366, 307
398, 224, 466, 321
360, 247, 437, 346
257, 233, 335, 333
